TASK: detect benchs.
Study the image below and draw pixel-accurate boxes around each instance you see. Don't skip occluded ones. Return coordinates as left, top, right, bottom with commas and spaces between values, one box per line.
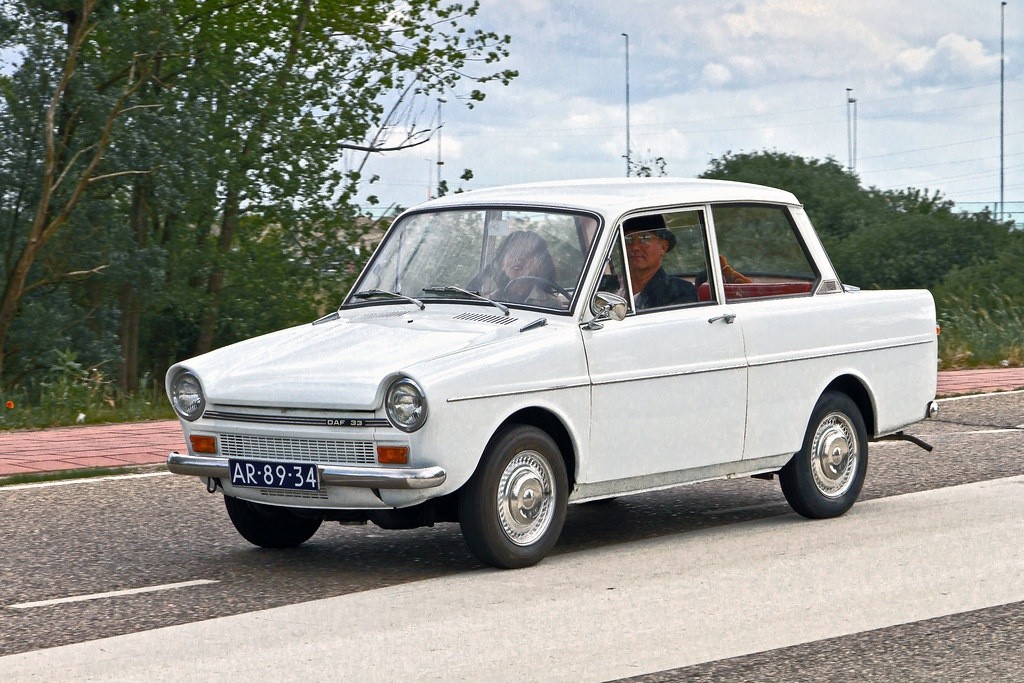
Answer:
692, 282, 813, 301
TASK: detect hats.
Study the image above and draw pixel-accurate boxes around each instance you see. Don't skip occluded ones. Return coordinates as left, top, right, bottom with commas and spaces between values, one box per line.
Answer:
615, 214, 677, 254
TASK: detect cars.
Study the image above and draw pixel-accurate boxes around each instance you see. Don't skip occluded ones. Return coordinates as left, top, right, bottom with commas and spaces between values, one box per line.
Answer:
165, 174, 942, 574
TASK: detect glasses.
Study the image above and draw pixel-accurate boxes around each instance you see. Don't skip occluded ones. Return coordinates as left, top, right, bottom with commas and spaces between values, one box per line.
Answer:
624, 234, 664, 244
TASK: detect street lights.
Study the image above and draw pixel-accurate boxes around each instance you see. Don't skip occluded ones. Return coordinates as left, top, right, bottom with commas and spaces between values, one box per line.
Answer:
438, 98, 448, 200
845, 87, 853, 172
847, 98, 858, 180
999, 1, 1010, 222
621, 32, 630, 177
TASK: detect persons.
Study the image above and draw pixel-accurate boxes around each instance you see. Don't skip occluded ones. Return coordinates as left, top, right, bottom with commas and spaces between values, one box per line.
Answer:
486, 231, 562, 308
617, 214, 700, 312
